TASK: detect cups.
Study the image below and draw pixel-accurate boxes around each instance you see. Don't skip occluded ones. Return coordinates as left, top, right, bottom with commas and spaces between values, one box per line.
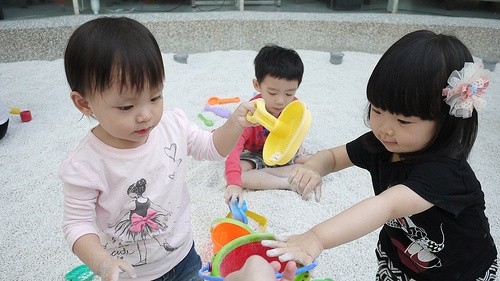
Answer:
11, 107, 32, 122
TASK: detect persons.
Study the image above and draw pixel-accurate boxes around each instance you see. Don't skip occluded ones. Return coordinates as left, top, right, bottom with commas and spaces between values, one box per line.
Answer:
261, 29, 499, 281
224, 42, 315, 208
59, 16, 260, 281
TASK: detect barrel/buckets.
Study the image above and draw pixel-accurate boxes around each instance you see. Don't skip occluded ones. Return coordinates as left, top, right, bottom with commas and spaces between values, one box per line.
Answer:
210, 211, 266, 255
198, 234, 305, 281
